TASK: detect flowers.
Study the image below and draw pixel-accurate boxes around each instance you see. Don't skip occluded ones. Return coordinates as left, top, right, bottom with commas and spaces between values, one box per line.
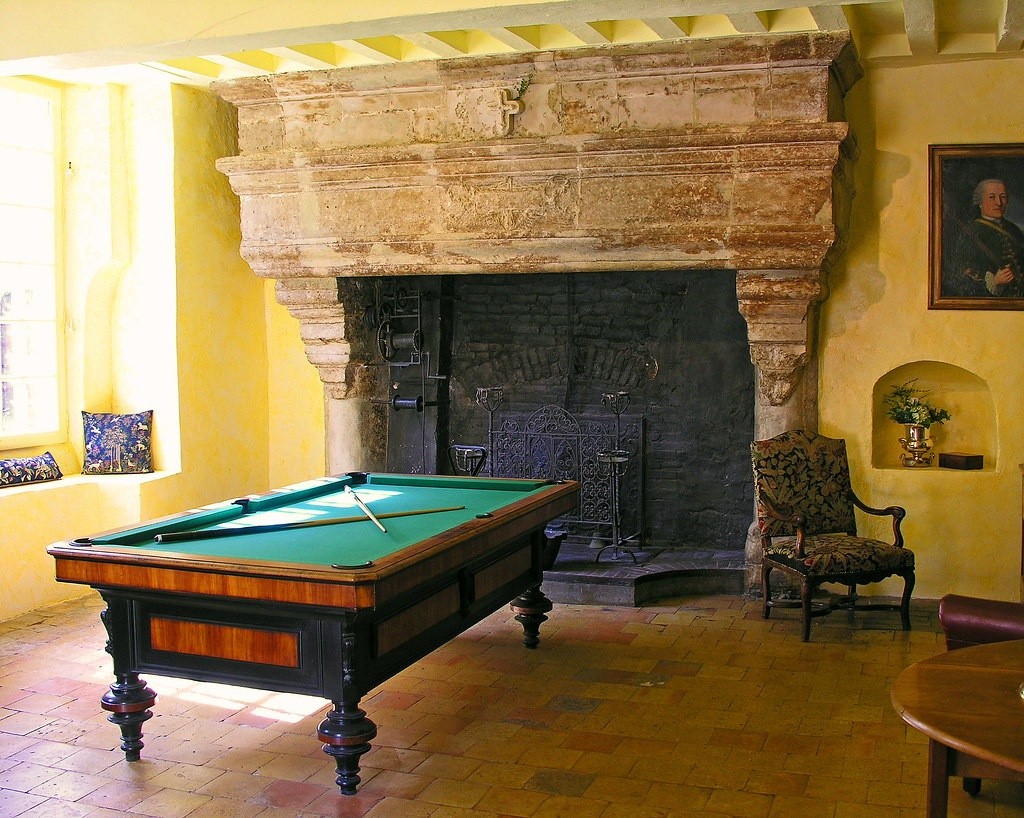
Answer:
880, 377, 954, 429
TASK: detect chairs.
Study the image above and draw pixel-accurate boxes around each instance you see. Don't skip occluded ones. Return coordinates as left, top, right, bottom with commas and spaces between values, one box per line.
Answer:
750, 429, 916, 641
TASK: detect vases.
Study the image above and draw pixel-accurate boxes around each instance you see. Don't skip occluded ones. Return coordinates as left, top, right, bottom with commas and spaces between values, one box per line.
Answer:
897, 423, 936, 468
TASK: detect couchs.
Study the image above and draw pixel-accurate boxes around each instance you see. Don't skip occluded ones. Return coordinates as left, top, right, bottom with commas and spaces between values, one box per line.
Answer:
938, 594, 1024, 652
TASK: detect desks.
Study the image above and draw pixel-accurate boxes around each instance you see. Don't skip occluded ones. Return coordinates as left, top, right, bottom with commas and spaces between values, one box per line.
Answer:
891, 639, 1024, 818
48, 471, 581, 795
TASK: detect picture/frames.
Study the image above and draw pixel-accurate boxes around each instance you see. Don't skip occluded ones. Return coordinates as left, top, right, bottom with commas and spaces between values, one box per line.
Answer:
927, 143, 1024, 311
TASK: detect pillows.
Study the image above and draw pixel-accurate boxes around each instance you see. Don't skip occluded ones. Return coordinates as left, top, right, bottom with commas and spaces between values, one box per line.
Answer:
81, 410, 155, 474
0, 450, 64, 489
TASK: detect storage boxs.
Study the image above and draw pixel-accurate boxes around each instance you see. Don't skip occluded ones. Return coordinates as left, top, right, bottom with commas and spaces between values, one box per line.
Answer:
939, 451, 983, 470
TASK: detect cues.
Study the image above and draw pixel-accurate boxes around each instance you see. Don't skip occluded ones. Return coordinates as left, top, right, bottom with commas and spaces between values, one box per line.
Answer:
343, 484, 386, 533
155, 505, 467, 543
